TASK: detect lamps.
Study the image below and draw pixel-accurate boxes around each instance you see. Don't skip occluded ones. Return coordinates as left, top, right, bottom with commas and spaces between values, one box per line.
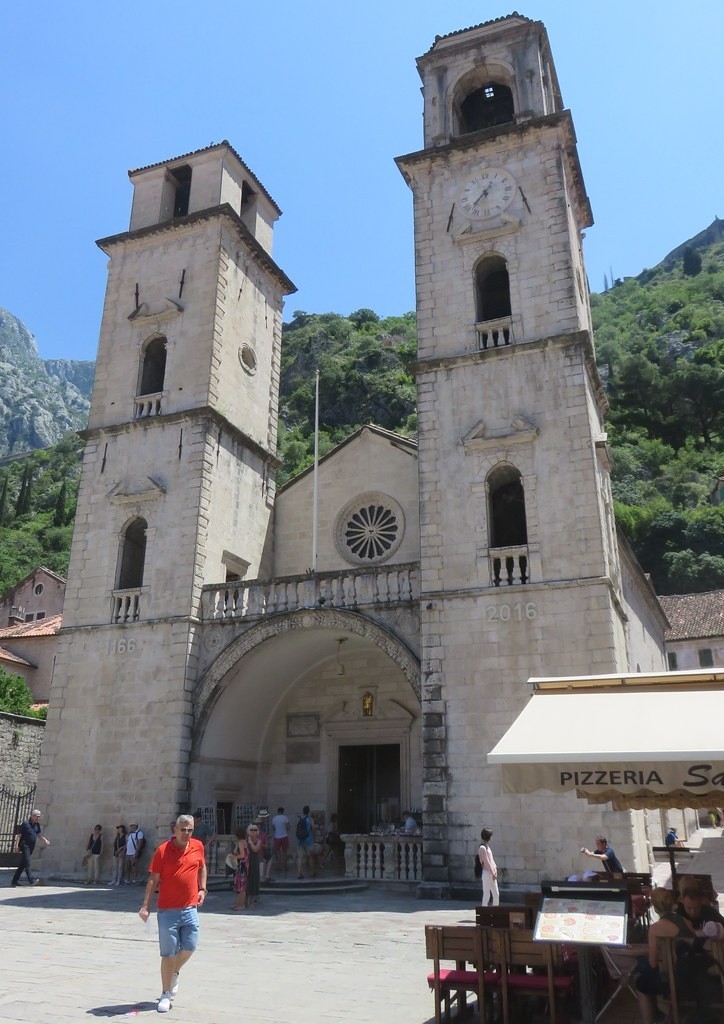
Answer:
337, 663, 344, 676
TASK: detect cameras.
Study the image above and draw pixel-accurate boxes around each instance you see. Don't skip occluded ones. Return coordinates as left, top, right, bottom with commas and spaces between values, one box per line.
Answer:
581, 849, 585, 852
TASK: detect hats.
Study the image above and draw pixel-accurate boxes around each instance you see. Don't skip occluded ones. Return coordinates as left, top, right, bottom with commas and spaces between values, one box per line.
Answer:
258, 809, 270, 817
225, 854, 237, 870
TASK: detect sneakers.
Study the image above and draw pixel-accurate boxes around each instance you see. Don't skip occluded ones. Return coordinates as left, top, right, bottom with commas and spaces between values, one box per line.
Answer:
158, 991, 170, 1012
168, 972, 180, 995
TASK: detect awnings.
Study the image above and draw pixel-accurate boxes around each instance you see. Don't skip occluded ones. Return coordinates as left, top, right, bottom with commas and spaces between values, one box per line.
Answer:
486, 681, 724, 811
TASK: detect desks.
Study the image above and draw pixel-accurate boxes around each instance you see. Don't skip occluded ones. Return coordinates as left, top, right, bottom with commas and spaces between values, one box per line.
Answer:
523, 892, 652, 936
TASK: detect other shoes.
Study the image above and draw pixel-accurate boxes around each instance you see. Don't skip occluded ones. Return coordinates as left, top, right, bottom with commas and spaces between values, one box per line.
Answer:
116, 881, 120, 885
108, 881, 116, 886
121, 882, 129, 886
11, 881, 21, 886
93, 880, 97, 884
297, 874, 304, 878
131, 882, 136, 887
30, 878, 39, 886
266, 878, 276, 882
231, 905, 253, 911
85, 881, 92, 884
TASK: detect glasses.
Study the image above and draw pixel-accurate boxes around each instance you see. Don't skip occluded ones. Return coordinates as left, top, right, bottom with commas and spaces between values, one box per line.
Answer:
249, 828, 258, 831
34, 816, 41, 819
176, 827, 194, 833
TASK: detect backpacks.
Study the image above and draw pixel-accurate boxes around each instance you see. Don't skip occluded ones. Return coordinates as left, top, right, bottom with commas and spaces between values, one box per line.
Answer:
475, 844, 487, 878
296, 816, 310, 840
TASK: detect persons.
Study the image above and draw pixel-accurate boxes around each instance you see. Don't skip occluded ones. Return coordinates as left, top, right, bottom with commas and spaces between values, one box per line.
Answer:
708, 807, 724, 829
584, 835, 623, 873
320, 814, 340, 869
11, 810, 51, 886
171, 811, 216, 904
228, 810, 275, 910
84, 824, 104, 885
402, 810, 417, 834
297, 806, 316, 879
139, 815, 208, 1012
478, 827, 499, 906
635, 875, 724, 1024
272, 808, 290, 871
666, 827, 682, 847
107, 823, 143, 886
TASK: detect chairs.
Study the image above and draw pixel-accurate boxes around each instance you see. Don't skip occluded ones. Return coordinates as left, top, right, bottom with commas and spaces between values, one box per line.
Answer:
424, 871, 724, 1024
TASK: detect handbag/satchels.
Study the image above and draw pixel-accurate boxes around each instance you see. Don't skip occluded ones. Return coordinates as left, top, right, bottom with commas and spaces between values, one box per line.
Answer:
326, 832, 340, 845
86, 849, 92, 857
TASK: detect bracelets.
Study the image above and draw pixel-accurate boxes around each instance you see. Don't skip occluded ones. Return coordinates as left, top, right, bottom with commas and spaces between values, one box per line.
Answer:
198, 888, 208, 896
143, 904, 150, 908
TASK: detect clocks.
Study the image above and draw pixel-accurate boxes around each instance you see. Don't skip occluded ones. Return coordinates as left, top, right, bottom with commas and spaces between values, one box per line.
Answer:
458, 167, 517, 221
